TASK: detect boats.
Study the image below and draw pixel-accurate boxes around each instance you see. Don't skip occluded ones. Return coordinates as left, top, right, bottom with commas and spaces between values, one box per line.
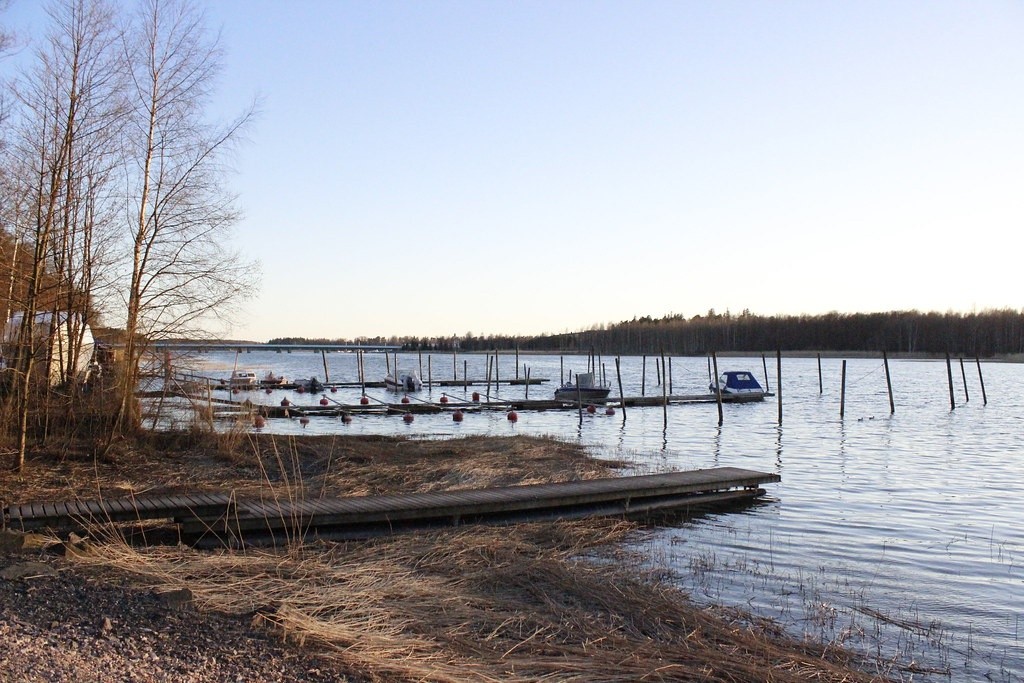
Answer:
229, 373, 256, 385
707, 370, 765, 399
554, 370, 612, 405
383, 368, 423, 393
260, 375, 282, 384
